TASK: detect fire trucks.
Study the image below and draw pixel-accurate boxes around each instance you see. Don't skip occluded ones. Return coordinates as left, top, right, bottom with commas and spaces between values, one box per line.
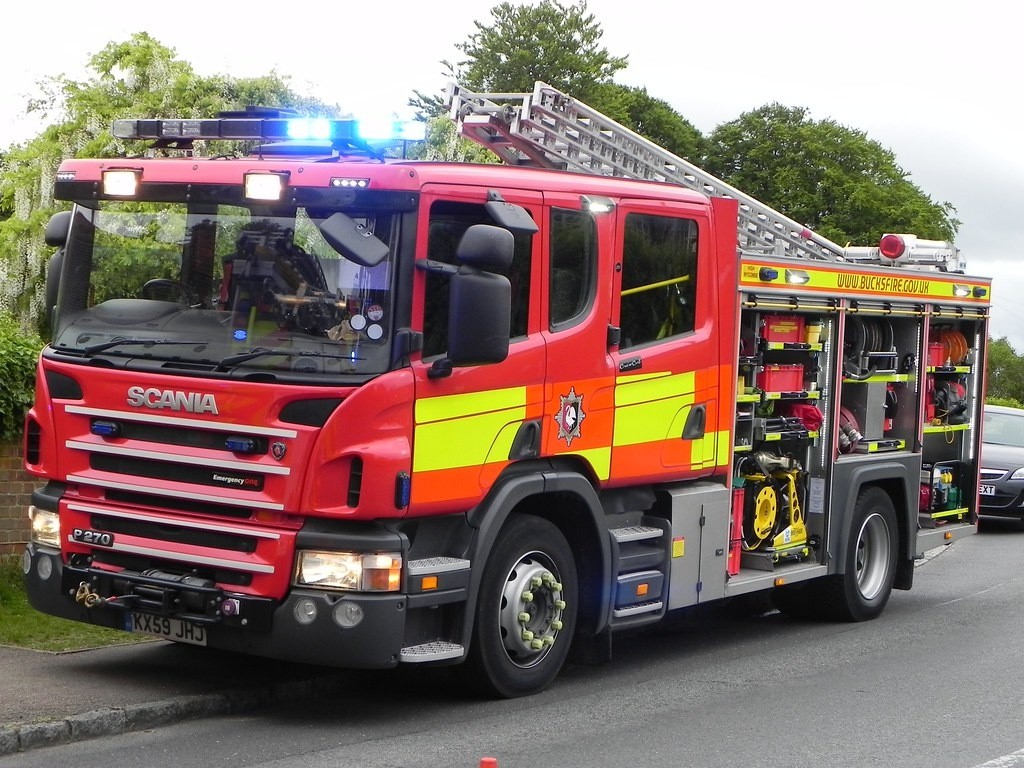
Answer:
19, 78, 993, 698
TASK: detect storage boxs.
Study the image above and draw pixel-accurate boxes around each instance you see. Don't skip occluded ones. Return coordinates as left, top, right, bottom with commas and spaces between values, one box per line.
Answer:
756, 364, 804, 391
929, 343, 945, 367
729, 487, 744, 539
761, 314, 804, 343
727, 540, 741, 575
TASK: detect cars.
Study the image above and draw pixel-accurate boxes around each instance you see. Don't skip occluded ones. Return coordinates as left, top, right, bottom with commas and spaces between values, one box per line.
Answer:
978, 404, 1024, 527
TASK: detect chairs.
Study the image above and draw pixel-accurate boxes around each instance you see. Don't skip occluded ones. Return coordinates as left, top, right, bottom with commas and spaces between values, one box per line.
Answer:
219, 225, 299, 317
